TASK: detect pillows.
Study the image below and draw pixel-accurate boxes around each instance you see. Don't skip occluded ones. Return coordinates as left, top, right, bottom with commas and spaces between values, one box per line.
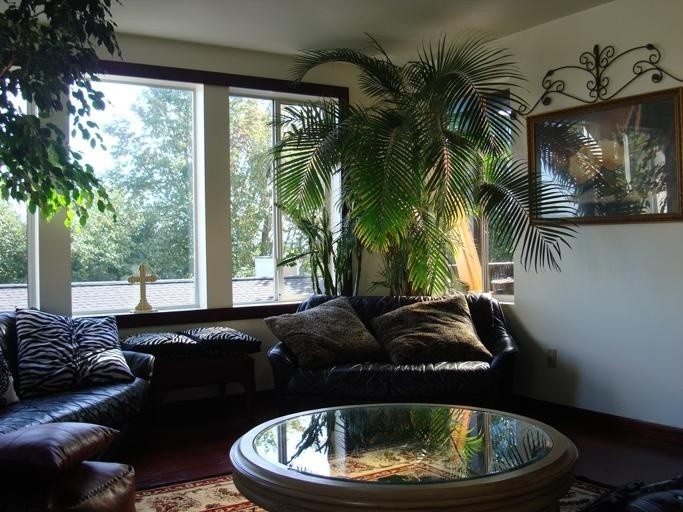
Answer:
0, 421, 120, 479
265, 295, 384, 372
118, 331, 200, 358
369, 294, 493, 365
14, 307, 135, 397
178, 325, 261, 355
0, 346, 21, 406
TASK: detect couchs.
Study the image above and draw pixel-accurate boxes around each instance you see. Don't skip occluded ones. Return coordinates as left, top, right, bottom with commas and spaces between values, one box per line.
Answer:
267, 292, 523, 407
0, 317, 155, 437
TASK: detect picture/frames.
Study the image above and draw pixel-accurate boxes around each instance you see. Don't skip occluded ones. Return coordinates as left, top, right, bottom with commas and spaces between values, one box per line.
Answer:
525, 86, 683, 226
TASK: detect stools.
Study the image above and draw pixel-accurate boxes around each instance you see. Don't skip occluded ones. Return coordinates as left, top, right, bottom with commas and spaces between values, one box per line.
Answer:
152, 351, 257, 419
0, 461, 136, 512
